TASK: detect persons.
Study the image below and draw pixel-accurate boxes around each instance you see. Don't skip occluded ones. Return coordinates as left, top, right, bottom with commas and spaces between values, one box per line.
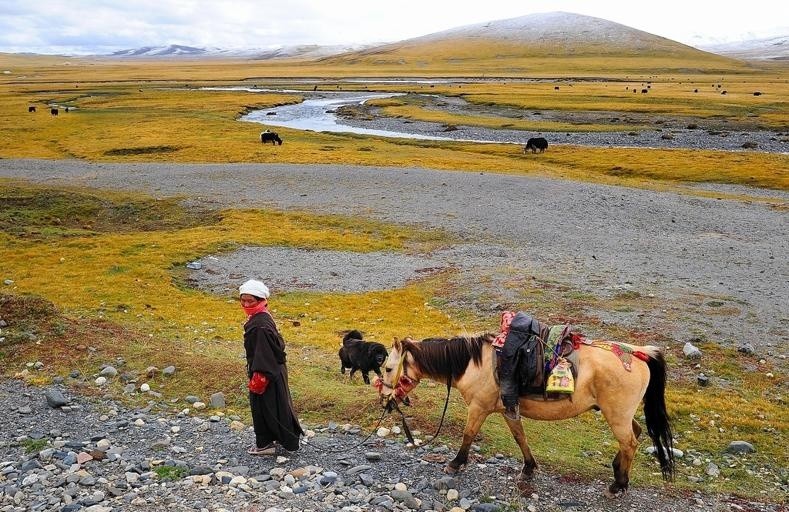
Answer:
238, 280, 302, 456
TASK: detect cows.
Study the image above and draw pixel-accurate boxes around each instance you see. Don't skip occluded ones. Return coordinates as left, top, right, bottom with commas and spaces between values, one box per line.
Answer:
260, 133, 282, 146
523, 138, 548, 155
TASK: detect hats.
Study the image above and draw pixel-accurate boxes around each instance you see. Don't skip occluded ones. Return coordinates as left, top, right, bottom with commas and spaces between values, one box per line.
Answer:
239, 280, 269, 300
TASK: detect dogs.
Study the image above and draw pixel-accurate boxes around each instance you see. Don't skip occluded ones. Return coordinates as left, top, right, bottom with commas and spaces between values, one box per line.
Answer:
339, 330, 388, 385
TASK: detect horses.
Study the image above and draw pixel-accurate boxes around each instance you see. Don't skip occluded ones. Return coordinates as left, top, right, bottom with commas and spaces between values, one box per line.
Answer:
378, 332, 676, 500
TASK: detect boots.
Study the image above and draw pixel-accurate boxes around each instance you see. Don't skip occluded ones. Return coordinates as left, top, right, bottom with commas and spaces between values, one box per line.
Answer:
248, 444, 276, 455
